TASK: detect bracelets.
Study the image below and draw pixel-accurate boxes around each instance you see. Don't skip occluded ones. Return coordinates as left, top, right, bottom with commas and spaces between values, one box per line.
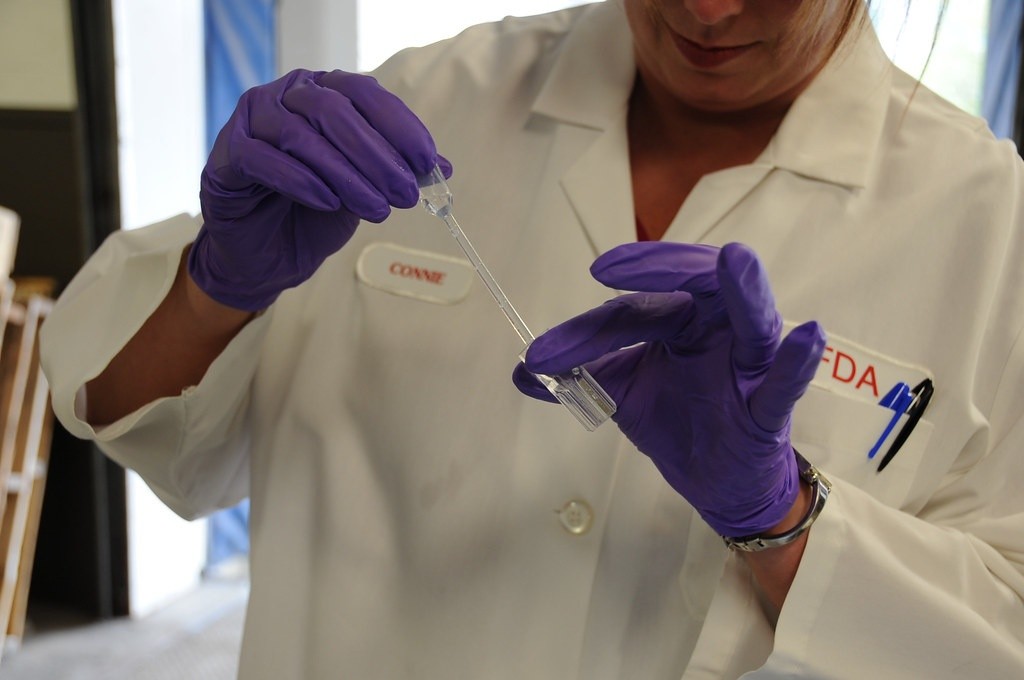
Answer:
723, 449, 832, 552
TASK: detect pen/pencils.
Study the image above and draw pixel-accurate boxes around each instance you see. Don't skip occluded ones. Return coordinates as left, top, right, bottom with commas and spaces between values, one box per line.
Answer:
867, 377, 935, 473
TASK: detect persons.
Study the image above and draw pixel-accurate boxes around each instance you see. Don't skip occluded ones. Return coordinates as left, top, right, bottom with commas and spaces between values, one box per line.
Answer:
37, 0, 1024, 680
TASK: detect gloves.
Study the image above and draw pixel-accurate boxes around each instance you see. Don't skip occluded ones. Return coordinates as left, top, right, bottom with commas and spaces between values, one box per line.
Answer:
185, 68, 454, 313
512, 241, 827, 538
